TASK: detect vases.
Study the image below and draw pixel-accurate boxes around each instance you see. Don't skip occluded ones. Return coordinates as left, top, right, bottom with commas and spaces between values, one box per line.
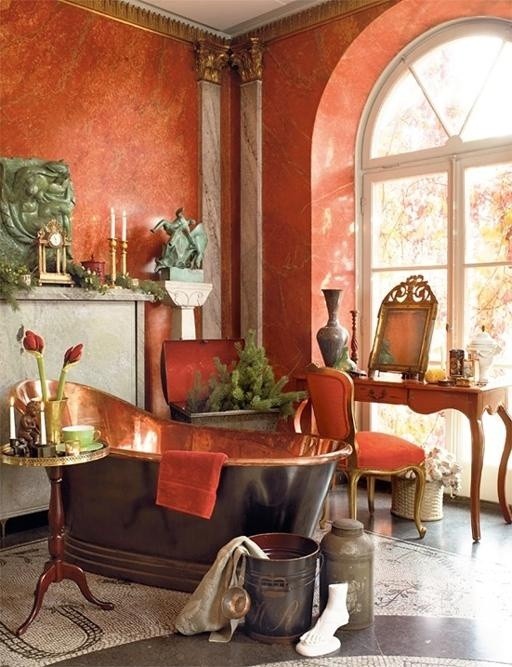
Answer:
314, 286, 349, 369
30, 397, 70, 450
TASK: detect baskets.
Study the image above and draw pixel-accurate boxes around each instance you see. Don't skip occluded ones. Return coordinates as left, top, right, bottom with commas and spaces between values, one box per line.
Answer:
390, 475, 444, 521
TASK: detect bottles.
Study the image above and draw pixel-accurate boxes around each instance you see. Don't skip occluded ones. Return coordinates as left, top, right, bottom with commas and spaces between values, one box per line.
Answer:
316, 289, 358, 375
319, 519, 375, 632
81, 252, 106, 285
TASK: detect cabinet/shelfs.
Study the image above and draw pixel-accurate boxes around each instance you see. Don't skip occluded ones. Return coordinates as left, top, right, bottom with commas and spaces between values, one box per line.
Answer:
0, 269, 156, 538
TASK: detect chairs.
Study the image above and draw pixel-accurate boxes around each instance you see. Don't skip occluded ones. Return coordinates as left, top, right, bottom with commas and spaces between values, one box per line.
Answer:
299, 362, 429, 541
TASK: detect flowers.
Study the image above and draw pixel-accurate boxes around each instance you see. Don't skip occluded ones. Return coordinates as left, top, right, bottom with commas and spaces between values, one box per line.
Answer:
401, 426, 462, 503
22, 327, 86, 402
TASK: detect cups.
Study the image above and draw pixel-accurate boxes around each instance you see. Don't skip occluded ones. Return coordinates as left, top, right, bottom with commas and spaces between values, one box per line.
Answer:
65, 440, 81, 456
61, 424, 102, 445
449, 349, 464, 374
465, 325, 505, 385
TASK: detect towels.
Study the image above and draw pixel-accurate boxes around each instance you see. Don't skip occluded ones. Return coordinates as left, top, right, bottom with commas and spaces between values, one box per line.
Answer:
151, 449, 229, 520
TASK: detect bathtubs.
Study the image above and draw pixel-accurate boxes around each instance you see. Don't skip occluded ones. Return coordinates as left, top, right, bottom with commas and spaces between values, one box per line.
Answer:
7, 377, 353, 595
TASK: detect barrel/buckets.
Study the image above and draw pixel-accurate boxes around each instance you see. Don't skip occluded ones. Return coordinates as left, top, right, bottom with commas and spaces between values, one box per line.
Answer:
243, 532, 321, 647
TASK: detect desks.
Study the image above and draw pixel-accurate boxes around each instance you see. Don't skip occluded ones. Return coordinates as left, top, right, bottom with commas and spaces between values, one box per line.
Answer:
1, 434, 119, 636
292, 370, 511, 548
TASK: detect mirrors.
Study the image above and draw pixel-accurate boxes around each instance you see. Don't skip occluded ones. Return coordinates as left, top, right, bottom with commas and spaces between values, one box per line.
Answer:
366, 273, 437, 384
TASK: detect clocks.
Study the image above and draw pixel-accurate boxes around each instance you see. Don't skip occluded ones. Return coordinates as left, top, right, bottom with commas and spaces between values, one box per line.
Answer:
33, 216, 74, 289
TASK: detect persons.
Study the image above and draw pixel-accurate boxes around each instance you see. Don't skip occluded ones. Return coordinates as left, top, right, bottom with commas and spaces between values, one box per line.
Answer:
17, 400, 42, 450
150, 206, 198, 248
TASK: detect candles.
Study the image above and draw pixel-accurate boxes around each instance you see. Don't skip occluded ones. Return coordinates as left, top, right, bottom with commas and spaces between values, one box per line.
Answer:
107, 206, 116, 238
122, 207, 127, 242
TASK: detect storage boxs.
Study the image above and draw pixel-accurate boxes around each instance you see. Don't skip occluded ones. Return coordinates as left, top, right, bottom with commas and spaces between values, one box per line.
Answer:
159, 335, 285, 438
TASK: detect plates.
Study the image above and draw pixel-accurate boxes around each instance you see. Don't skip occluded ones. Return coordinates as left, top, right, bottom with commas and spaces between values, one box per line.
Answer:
56, 441, 103, 453
